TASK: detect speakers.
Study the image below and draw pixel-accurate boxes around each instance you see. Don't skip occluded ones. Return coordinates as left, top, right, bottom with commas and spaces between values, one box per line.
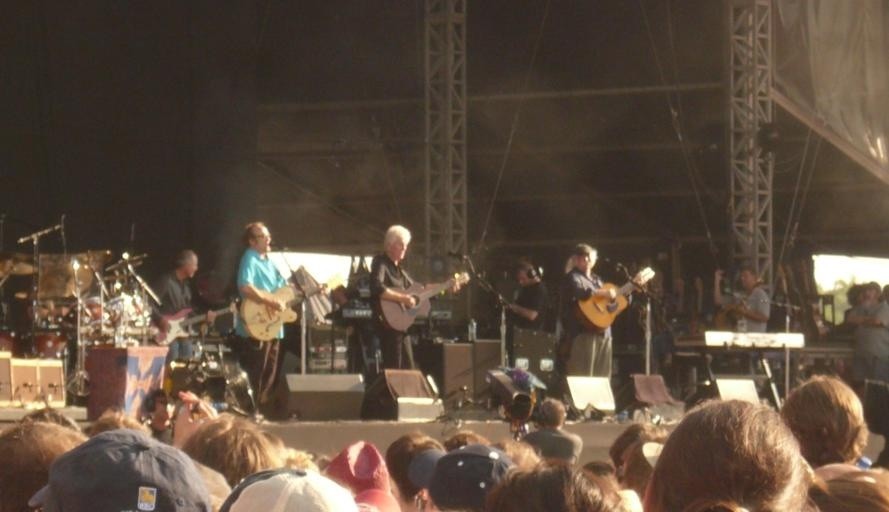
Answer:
269, 370, 367, 419
359, 368, 444, 422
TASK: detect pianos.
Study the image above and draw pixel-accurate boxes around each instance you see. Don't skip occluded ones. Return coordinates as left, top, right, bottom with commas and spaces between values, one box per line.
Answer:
672, 327, 807, 354
322, 302, 454, 325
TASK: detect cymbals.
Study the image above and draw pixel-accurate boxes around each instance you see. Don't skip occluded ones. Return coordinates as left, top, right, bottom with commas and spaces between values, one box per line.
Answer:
101, 252, 149, 282
0, 251, 40, 277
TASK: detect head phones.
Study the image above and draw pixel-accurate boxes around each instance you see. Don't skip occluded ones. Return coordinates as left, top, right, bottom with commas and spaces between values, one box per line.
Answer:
526, 267, 539, 279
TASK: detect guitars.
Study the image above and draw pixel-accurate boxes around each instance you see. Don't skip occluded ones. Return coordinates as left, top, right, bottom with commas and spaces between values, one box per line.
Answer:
152, 304, 241, 347
240, 279, 331, 341
708, 277, 767, 333
378, 269, 473, 333
573, 267, 658, 332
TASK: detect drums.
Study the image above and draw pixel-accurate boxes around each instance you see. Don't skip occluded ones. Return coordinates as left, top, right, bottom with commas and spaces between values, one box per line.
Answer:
70, 295, 105, 327
106, 294, 136, 321
21, 327, 72, 360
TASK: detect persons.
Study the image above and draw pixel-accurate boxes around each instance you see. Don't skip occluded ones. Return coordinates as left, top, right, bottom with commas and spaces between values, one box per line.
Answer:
233, 222, 308, 424
150, 247, 216, 324
350, 223, 462, 417
1, 375, 888, 512
558, 242, 646, 376
502, 258, 554, 401
650, 265, 889, 385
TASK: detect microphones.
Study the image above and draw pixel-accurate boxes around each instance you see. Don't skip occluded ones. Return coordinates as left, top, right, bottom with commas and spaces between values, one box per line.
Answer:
447, 248, 468, 265
17, 223, 63, 248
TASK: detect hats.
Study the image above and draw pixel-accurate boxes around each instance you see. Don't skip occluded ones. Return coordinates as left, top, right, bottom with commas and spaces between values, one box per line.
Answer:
325, 442, 389, 492
407, 444, 514, 511
354, 490, 401, 512
28, 429, 210, 512
218, 468, 356, 511
